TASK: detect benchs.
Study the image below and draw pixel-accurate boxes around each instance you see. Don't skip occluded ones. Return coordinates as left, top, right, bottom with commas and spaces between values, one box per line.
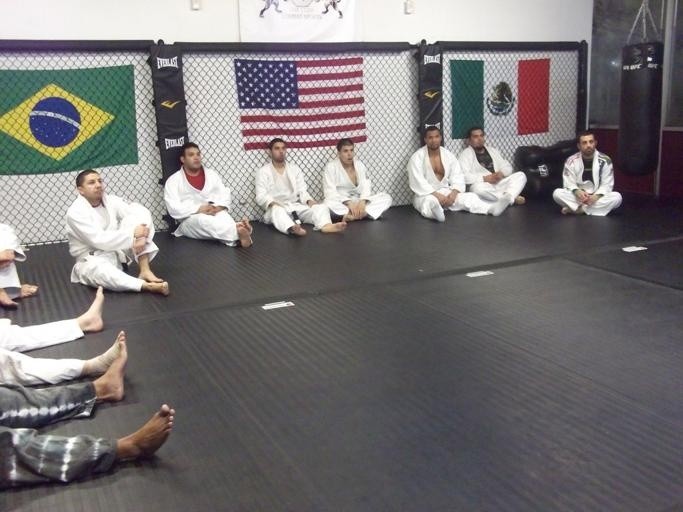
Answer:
448, 56, 552, 142
233, 54, 368, 152
0, 64, 138, 174
236, 0, 355, 45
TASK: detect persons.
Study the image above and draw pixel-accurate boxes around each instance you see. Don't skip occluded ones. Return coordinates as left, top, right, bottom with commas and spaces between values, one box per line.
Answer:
162, 142, 253, 249
550, 128, 625, 216
253, 138, 347, 236
0, 285, 126, 387
0, 341, 176, 490
62, 168, 171, 297
321, 137, 393, 223
456, 128, 528, 207
405, 126, 513, 223
0, 223, 39, 308
